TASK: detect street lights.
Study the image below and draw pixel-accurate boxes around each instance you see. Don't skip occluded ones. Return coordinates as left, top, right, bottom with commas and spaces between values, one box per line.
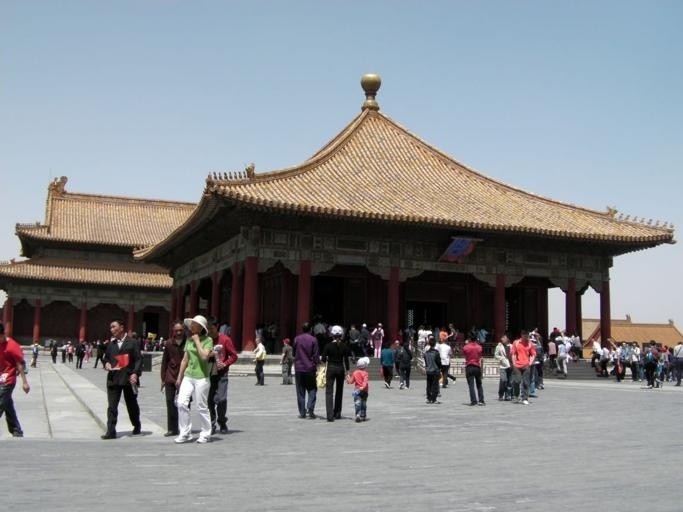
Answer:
316, 365, 326, 389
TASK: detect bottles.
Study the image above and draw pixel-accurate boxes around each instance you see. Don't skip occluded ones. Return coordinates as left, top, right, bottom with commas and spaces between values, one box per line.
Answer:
184, 314, 209, 336
331, 326, 344, 336
357, 356, 370, 369
283, 339, 290, 344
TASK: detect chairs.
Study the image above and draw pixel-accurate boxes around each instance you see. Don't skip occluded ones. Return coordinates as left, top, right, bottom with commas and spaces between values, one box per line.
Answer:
164, 430, 211, 443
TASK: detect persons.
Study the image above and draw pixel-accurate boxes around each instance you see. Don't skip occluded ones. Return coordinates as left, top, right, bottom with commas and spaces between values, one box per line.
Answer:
205, 317, 238, 435
49, 339, 110, 369
175, 317, 213, 443
160, 319, 187, 437
423, 334, 442, 404
31, 343, 39, 368
0, 322, 30, 437
322, 325, 350, 421
292, 322, 319, 418
252, 337, 266, 386
255, 320, 279, 354
380, 339, 412, 389
280, 338, 293, 385
314, 319, 326, 354
220, 321, 231, 336
494, 329, 544, 405
547, 327, 581, 378
100, 320, 143, 439
404, 323, 488, 354
349, 322, 384, 358
435, 338, 457, 388
132, 332, 166, 351
590, 336, 683, 389
347, 356, 370, 423
462, 335, 486, 406
